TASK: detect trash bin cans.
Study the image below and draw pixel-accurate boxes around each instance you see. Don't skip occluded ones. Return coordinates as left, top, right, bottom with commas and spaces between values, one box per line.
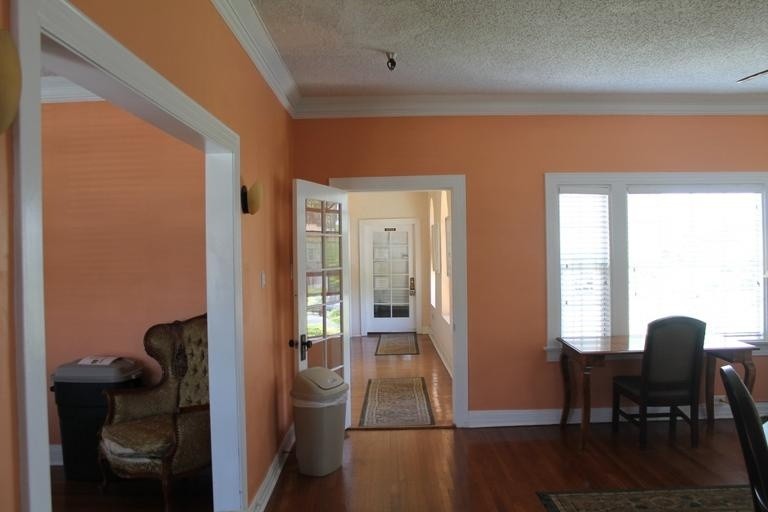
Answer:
291, 366, 349, 476
50, 357, 143, 482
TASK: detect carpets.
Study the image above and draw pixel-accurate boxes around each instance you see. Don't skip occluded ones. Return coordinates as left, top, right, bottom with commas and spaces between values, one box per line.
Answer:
535, 486, 754, 512
359, 378, 435, 428
375, 331, 419, 355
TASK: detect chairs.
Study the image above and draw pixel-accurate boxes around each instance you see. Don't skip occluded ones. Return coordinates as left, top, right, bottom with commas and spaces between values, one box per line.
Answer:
613, 315, 704, 451
719, 365, 768, 511
98, 311, 213, 512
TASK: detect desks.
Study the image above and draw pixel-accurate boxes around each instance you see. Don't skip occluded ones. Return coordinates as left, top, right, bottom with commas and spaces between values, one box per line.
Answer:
557, 337, 760, 446
